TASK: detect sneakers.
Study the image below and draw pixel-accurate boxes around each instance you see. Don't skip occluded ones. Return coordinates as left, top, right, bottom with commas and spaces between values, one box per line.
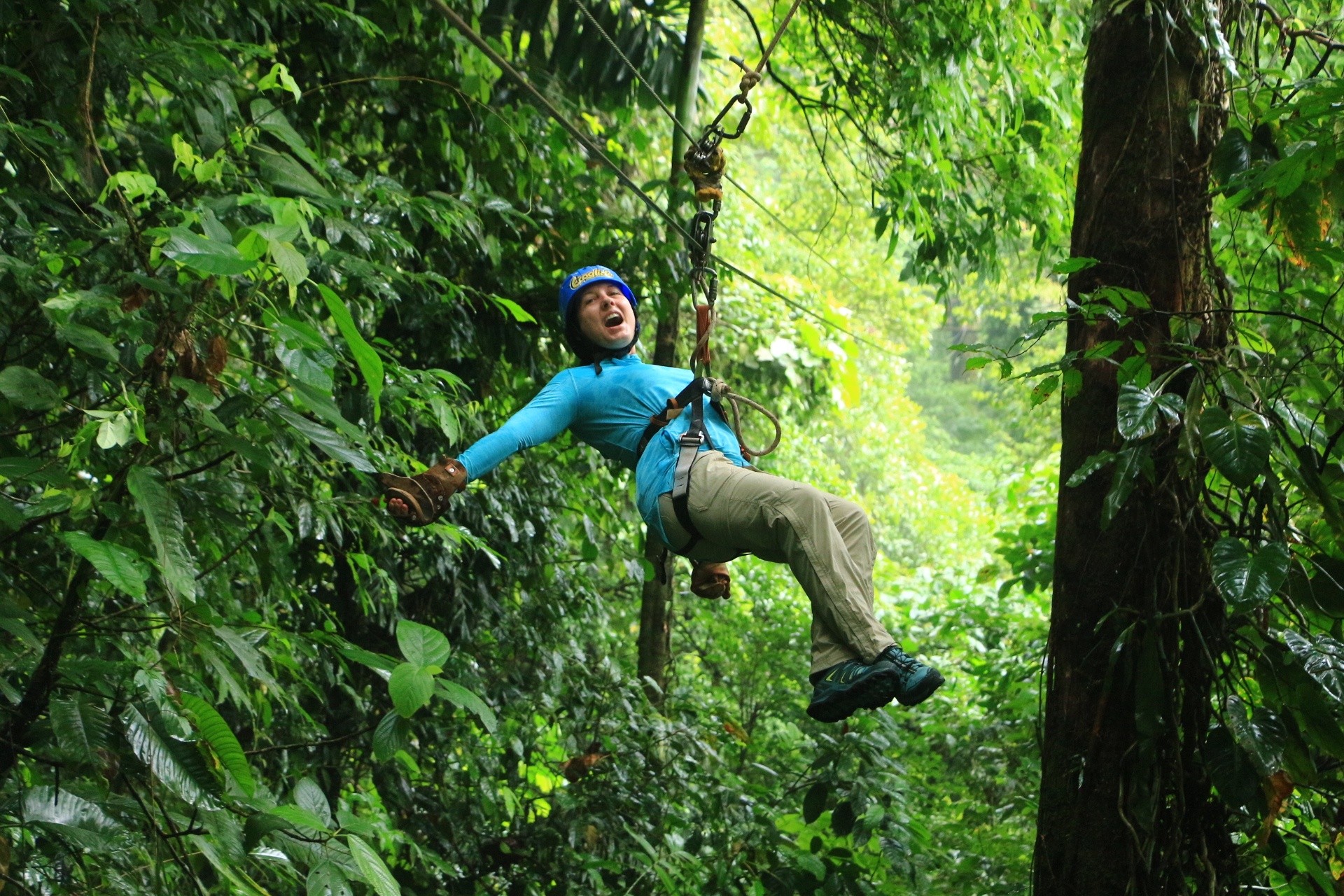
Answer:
805, 659, 902, 722
872, 641, 947, 707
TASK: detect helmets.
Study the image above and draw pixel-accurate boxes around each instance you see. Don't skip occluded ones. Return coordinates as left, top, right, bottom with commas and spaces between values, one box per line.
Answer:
559, 264, 638, 362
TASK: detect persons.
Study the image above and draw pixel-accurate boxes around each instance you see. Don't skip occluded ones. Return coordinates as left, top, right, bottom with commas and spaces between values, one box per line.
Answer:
388, 266, 944, 723
